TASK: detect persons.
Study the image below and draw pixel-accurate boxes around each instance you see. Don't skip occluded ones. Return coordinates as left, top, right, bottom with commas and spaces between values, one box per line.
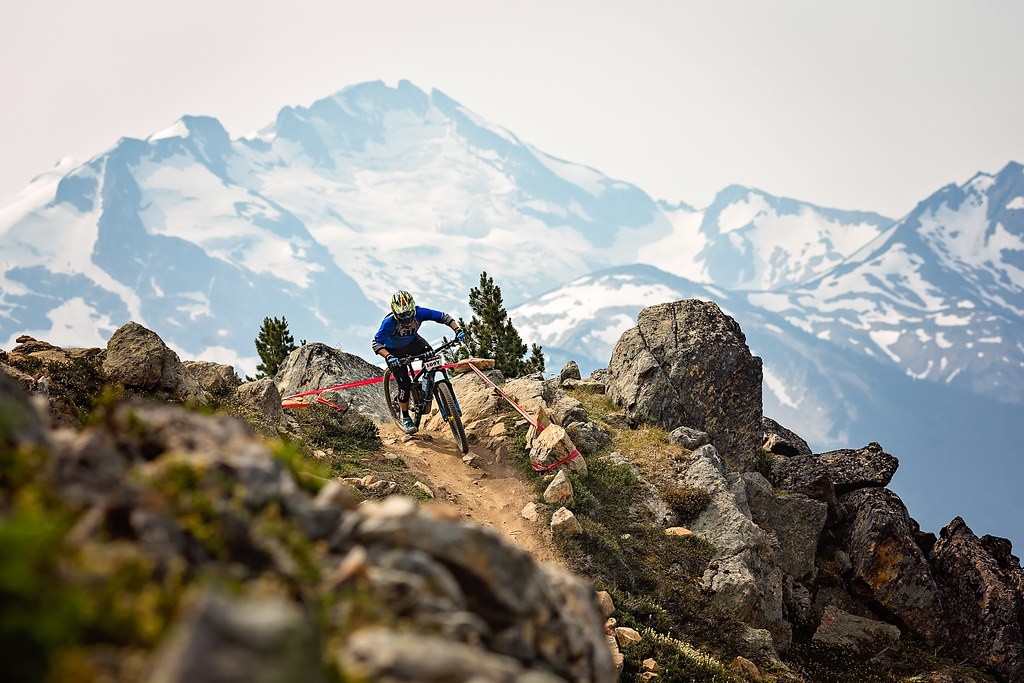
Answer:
372, 290, 466, 434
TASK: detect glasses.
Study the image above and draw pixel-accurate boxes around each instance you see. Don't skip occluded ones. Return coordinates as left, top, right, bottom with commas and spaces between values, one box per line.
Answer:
397, 311, 415, 319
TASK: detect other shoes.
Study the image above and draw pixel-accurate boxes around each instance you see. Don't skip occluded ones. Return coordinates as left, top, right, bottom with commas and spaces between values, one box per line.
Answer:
403, 416, 418, 432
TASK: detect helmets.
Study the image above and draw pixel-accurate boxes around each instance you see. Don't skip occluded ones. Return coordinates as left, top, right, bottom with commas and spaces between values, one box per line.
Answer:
391, 290, 416, 326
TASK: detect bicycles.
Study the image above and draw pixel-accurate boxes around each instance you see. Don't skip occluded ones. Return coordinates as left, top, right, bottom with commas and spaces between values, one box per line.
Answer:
383, 337, 471, 454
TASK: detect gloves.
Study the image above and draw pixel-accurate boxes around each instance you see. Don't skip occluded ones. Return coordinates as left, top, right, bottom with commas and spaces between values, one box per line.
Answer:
454, 327, 466, 342
385, 354, 399, 369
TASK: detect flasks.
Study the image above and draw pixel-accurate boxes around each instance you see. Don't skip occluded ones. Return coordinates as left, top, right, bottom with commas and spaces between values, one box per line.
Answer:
422, 372, 429, 391
